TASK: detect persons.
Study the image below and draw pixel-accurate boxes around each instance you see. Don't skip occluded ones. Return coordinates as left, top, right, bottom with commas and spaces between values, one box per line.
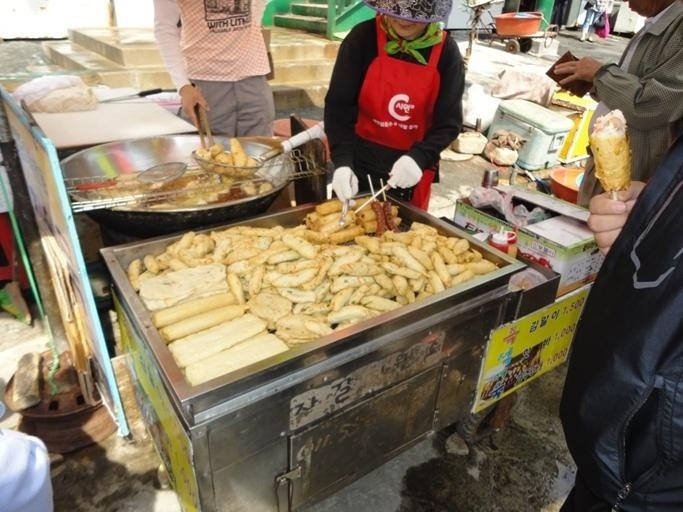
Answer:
152, 0, 277, 143
321, 2, 465, 228
550, 0, 682, 208
580, 0, 613, 44
550, 138, 681, 511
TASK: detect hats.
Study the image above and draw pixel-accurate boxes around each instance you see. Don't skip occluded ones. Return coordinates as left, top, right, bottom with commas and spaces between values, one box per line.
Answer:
363, 1, 453, 24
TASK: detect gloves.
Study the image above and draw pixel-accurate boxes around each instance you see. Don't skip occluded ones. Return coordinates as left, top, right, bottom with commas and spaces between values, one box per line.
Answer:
324, 164, 360, 207
386, 153, 423, 189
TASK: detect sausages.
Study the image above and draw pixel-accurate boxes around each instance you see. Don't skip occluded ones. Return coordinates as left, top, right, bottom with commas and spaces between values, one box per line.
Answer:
371, 201, 387, 236
384, 200, 401, 233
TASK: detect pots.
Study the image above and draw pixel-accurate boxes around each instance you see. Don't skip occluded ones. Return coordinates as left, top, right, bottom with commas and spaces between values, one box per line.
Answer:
59, 134, 296, 240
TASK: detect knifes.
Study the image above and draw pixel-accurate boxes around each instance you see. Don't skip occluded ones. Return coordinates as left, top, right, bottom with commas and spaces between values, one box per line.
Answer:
100, 89, 163, 104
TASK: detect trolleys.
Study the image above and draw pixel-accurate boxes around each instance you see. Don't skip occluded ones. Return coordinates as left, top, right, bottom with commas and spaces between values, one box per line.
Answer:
480, 11, 559, 53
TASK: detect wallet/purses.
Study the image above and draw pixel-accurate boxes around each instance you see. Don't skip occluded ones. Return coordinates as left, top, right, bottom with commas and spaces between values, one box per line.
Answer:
546, 50, 596, 99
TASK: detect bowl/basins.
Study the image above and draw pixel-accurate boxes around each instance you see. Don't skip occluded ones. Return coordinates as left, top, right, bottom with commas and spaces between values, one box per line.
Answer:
551, 168, 586, 204
494, 12, 541, 36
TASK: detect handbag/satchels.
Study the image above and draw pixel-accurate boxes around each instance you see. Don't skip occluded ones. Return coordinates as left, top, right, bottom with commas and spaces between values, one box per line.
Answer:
593, 13, 606, 29
594, 10, 610, 39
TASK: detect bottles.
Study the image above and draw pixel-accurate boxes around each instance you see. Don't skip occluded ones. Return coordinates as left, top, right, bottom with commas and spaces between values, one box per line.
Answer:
490, 234, 509, 253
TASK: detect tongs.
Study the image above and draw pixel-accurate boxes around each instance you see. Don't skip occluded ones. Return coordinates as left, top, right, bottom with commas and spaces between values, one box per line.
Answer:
192, 80, 216, 151
